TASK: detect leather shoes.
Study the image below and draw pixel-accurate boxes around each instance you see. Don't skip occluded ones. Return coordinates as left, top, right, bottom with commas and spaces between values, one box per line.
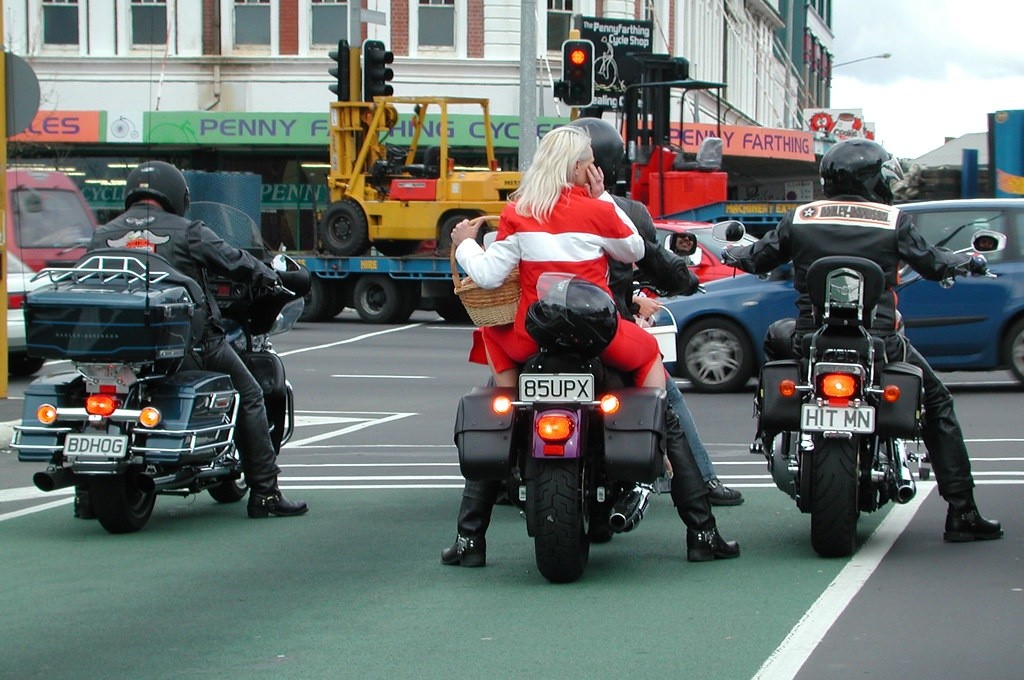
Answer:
440, 535, 486, 567
246, 488, 308, 518
943, 511, 1004, 543
685, 526, 739, 562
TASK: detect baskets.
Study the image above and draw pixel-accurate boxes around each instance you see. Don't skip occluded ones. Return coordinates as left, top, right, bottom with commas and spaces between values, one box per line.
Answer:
451, 215, 520, 328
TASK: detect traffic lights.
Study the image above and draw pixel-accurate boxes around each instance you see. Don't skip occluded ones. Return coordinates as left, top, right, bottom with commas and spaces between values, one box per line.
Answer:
360, 39, 395, 105
562, 39, 596, 109
328, 38, 352, 99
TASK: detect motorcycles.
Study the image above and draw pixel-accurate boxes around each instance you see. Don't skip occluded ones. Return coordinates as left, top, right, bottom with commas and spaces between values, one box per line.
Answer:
509, 235, 671, 582
14, 203, 298, 537
708, 216, 1009, 564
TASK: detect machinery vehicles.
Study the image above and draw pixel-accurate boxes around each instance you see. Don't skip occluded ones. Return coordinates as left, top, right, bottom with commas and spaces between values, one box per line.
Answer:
257, 93, 532, 258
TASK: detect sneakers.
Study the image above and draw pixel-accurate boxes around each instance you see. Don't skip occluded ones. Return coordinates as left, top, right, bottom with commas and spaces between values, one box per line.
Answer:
705, 479, 744, 505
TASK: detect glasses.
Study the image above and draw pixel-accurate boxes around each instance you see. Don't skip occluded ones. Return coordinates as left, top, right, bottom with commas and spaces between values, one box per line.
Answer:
679, 234, 694, 241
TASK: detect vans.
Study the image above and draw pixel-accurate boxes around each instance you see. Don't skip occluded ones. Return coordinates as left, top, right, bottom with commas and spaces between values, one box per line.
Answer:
3, 167, 102, 352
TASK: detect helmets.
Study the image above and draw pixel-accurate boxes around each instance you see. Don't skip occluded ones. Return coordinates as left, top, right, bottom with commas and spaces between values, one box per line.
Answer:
975, 235, 998, 251
818, 140, 895, 203
669, 233, 697, 254
124, 162, 189, 217
570, 117, 624, 190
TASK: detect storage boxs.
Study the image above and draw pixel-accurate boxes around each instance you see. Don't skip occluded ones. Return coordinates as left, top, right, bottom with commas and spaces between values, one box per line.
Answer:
758, 358, 801, 430
146, 370, 235, 458
25, 277, 193, 364
876, 361, 925, 438
453, 385, 519, 483
602, 385, 668, 484
18, 370, 84, 461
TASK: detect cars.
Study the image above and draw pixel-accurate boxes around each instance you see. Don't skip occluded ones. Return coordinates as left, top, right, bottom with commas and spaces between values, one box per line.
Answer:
640, 218, 767, 305
645, 199, 1024, 384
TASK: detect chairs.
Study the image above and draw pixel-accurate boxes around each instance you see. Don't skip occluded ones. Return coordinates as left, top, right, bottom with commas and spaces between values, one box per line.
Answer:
674, 137, 722, 172
409, 143, 451, 179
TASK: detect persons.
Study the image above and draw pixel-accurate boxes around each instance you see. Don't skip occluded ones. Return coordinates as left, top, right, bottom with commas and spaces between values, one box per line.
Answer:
439, 117, 740, 567
87, 159, 309, 519
451, 124, 745, 507
721, 137, 1004, 543
670, 233, 697, 264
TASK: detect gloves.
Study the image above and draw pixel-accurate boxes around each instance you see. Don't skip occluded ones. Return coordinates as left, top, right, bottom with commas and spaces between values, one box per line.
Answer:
966, 250, 989, 275
721, 245, 733, 259
